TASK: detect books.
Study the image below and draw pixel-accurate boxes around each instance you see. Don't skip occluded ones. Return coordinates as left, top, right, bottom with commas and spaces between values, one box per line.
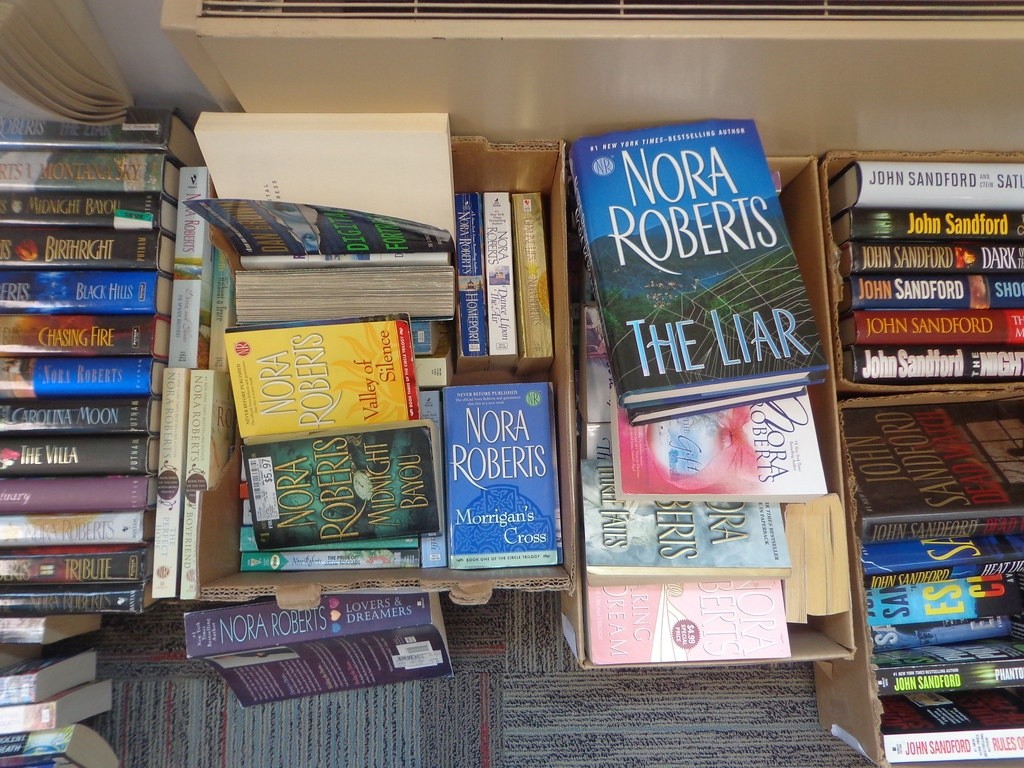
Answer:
1, 110, 1024, 768
569, 119, 831, 406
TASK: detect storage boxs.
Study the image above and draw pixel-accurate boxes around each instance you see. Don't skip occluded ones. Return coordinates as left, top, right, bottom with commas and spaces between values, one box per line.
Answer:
817, 136, 1024, 768
162, 133, 578, 611
561, 155, 856, 672
